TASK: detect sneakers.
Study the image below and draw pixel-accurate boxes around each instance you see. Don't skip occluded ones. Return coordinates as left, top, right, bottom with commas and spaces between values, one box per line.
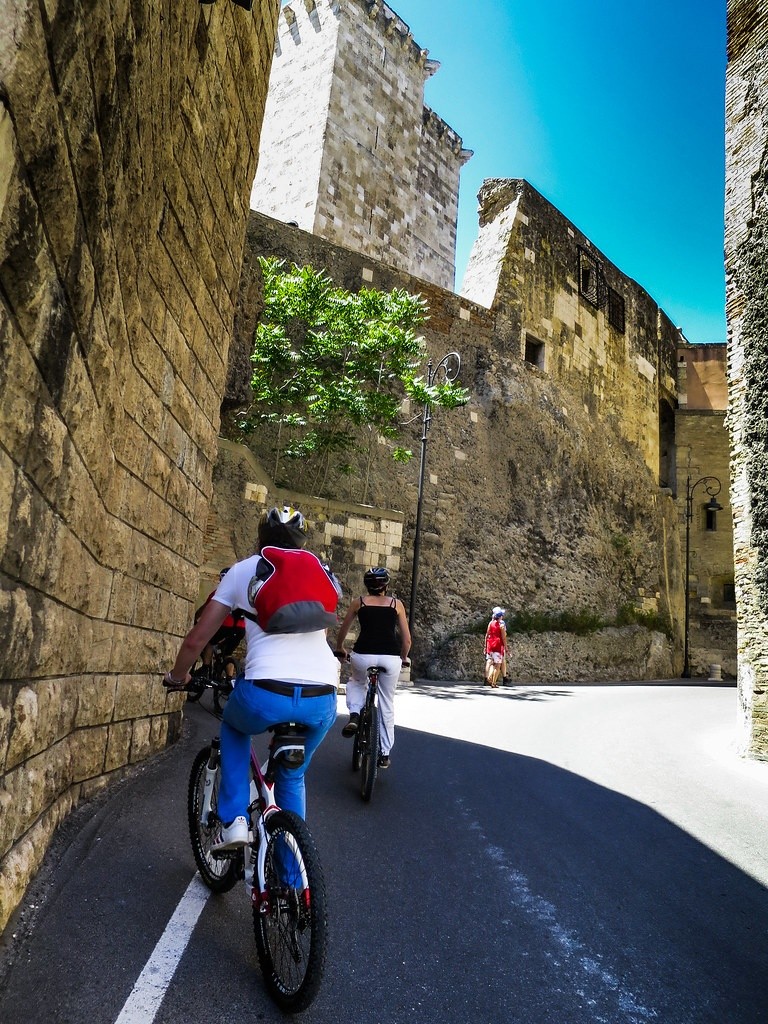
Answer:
209, 815, 249, 853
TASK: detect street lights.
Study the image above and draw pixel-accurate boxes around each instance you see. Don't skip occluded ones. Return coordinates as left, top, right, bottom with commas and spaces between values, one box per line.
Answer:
410, 350, 463, 639
681, 475, 727, 679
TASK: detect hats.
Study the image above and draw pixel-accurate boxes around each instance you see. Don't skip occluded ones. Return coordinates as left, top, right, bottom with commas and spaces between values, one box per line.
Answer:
494, 611, 504, 618
492, 606, 505, 618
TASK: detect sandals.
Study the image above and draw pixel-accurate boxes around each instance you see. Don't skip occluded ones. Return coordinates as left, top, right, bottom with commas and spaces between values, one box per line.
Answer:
487, 677, 499, 688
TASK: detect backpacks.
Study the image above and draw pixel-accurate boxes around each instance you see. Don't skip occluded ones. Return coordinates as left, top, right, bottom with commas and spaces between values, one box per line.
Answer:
238, 545, 341, 635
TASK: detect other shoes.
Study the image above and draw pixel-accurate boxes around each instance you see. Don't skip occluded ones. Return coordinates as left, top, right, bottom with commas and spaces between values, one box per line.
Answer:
377, 758, 391, 768
194, 663, 211, 677
503, 680, 513, 687
483, 677, 492, 686
342, 718, 360, 738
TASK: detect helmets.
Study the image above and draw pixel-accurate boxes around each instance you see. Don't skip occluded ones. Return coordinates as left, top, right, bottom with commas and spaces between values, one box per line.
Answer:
219, 568, 230, 581
363, 567, 391, 591
259, 505, 307, 549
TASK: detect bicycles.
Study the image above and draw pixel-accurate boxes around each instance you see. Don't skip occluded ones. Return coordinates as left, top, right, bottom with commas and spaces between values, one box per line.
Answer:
185, 622, 242, 716
162, 679, 328, 1014
330, 650, 410, 806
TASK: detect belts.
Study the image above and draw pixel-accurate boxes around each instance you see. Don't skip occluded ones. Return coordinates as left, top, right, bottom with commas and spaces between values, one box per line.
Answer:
247, 677, 335, 698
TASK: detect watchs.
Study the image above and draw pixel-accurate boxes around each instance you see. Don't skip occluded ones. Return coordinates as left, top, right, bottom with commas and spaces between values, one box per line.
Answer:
168, 669, 186, 686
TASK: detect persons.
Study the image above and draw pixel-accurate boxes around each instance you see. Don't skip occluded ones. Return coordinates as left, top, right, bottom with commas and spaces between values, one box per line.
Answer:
337, 567, 411, 769
483, 606, 509, 688
164, 506, 343, 892
